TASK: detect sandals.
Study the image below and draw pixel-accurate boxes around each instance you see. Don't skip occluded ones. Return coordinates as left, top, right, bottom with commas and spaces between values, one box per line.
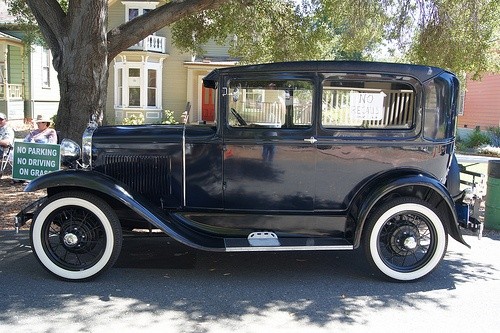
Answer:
13, 179, 30, 185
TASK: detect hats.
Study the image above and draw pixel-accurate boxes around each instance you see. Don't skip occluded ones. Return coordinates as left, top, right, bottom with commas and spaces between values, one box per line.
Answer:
31, 113, 53, 122
0, 111, 6, 119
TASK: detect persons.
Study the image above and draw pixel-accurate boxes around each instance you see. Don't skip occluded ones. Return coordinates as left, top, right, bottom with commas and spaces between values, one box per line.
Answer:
0, 112, 14, 178
10, 114, 57, 186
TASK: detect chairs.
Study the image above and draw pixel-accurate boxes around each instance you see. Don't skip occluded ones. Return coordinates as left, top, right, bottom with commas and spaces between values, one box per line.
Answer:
60, 138, 81, 170
0, 145, 13, 176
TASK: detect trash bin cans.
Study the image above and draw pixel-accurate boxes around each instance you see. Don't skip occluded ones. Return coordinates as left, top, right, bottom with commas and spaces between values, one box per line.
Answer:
484, 159, 500, 230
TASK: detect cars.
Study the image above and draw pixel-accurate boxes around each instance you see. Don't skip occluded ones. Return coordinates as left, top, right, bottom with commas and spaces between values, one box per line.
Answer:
13, 59, 483, 285
